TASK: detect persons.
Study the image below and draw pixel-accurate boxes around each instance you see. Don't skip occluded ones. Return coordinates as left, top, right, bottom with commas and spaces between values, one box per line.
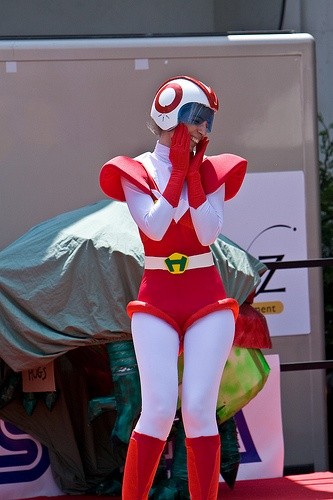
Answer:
99, 74, 251, 500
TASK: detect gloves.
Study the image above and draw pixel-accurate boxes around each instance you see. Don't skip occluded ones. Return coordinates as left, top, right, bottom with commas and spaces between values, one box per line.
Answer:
186, 136, 209, 210
162, 123, 190, 208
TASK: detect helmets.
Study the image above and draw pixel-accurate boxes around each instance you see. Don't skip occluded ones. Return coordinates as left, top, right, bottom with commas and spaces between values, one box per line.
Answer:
150, 75, 219, 130
